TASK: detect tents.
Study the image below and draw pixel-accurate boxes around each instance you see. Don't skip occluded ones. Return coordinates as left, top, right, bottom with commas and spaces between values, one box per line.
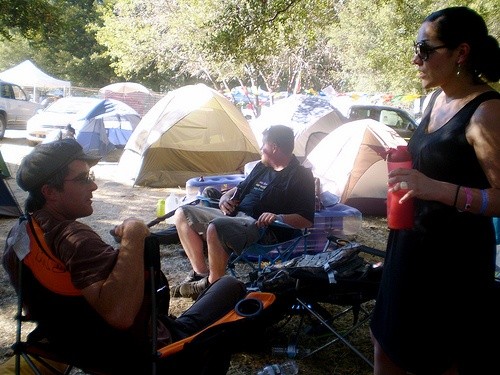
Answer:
112, 82, 262, 190
98, 83, 152, 114
303, 117, 408, 219
27, 96, 141, 162
0, 59, 70, 101
249, 94, 342, 164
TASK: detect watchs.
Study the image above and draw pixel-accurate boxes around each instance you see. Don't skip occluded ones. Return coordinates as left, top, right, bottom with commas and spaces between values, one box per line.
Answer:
457, 186, 472, 213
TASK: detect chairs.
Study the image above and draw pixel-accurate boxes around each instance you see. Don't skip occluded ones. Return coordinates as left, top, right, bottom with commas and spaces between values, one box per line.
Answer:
14, 197, 386, 375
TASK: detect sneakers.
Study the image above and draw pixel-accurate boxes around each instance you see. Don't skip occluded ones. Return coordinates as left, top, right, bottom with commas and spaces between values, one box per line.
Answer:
168, 269, 204, 297
179, 276, 209, 298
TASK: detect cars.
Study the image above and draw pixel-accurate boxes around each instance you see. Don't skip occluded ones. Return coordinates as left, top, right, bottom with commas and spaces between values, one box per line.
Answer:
27, 97, 141, 144
348, 104, 417, 143
0, 79, 45, 140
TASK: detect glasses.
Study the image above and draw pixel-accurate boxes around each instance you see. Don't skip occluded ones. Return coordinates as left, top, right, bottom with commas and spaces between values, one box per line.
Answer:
413, 41, 458, 61
62, 171, 95, 185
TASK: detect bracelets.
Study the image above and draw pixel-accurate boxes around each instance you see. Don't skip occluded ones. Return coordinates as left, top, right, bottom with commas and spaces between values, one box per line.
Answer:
454, 185, 461, 207
480, 189, 488, 214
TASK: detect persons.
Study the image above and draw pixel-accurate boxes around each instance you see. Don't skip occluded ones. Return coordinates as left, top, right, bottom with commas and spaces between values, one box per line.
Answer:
16, 138, 246, 375
174, 126, 315, 297
368, 6, 500, 375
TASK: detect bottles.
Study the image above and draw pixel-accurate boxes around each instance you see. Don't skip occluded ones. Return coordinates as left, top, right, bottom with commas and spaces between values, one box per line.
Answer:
270, 345, 311, 357
258, 358, 299, 375
383, 145, 413, 229
157, 199, 165, 218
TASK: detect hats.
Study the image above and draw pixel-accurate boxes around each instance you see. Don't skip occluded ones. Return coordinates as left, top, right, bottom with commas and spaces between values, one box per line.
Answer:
17, 138, 103, 192
